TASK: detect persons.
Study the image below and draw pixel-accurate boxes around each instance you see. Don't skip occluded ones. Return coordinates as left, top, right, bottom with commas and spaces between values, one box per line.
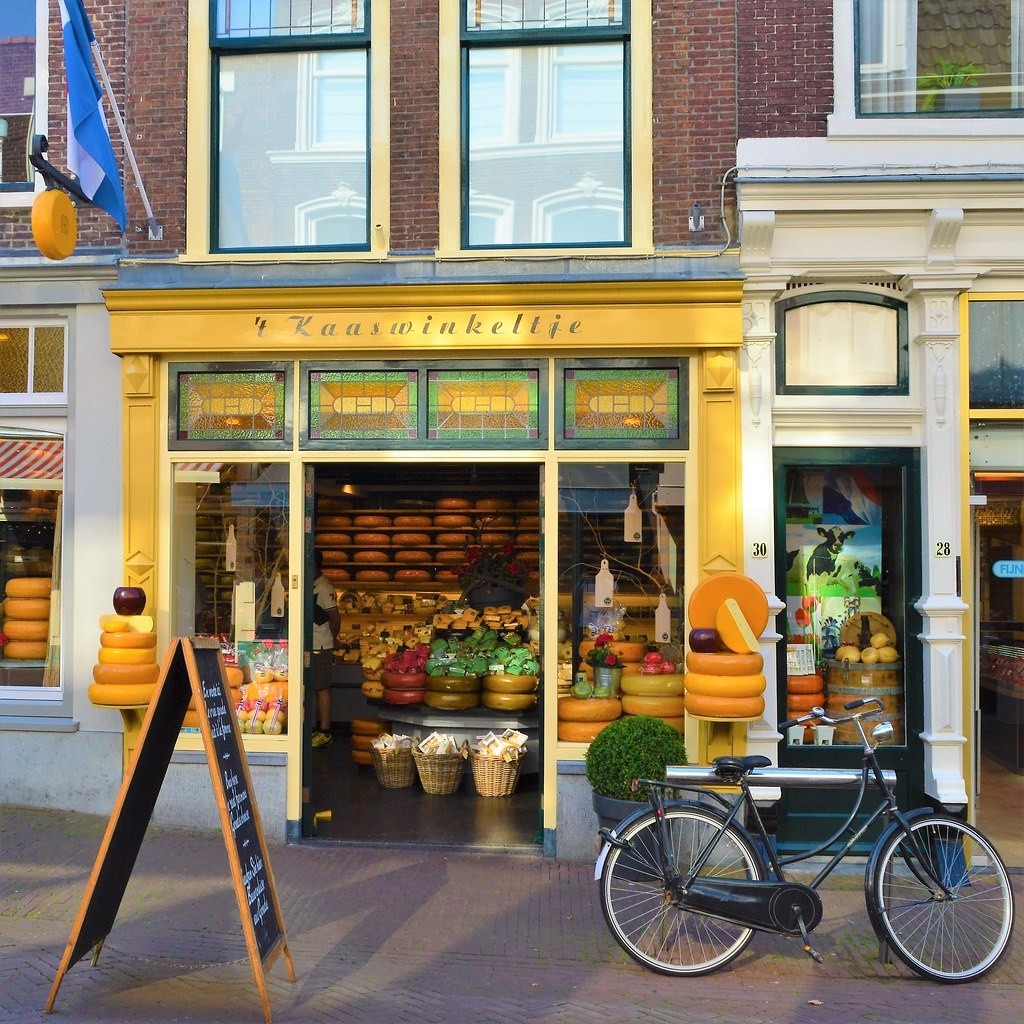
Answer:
310, 550, 342, 748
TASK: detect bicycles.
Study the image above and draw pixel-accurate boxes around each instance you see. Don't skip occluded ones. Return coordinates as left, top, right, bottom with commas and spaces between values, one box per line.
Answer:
595, 697, 1017, 985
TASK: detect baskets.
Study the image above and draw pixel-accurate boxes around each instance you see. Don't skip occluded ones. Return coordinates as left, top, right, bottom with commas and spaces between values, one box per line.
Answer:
367, 733, 415, 789
412, 735, 468, 795
470, 747, 526, 795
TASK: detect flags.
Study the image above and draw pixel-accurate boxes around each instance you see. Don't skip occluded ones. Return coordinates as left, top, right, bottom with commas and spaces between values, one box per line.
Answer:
57, 0, 128, 231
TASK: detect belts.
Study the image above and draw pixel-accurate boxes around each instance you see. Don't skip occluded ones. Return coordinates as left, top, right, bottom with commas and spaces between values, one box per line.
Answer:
313, 650, 323, 655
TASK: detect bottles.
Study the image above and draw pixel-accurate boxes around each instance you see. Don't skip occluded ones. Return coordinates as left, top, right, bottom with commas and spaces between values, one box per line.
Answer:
575, 670, 587, 683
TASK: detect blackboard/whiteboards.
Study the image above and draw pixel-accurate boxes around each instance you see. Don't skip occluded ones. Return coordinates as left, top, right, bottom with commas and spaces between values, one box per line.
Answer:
179, 634, 289, 976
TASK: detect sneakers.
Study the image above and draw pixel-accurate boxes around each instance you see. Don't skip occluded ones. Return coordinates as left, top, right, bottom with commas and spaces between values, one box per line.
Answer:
312, 730, 334, 748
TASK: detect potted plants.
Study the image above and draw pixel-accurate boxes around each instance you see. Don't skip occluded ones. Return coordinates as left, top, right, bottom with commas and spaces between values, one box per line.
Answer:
586, 715, 688, 882
917, 38, 985, 111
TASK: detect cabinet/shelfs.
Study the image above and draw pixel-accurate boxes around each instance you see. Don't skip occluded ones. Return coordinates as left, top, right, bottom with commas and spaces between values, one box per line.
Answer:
196, 509, 684, 606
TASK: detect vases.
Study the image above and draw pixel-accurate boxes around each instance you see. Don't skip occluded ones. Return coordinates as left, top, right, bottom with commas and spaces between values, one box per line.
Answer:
467, 582, 523, 610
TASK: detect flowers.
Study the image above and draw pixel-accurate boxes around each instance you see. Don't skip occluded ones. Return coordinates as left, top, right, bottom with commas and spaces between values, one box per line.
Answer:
451, 510, 528, 588
